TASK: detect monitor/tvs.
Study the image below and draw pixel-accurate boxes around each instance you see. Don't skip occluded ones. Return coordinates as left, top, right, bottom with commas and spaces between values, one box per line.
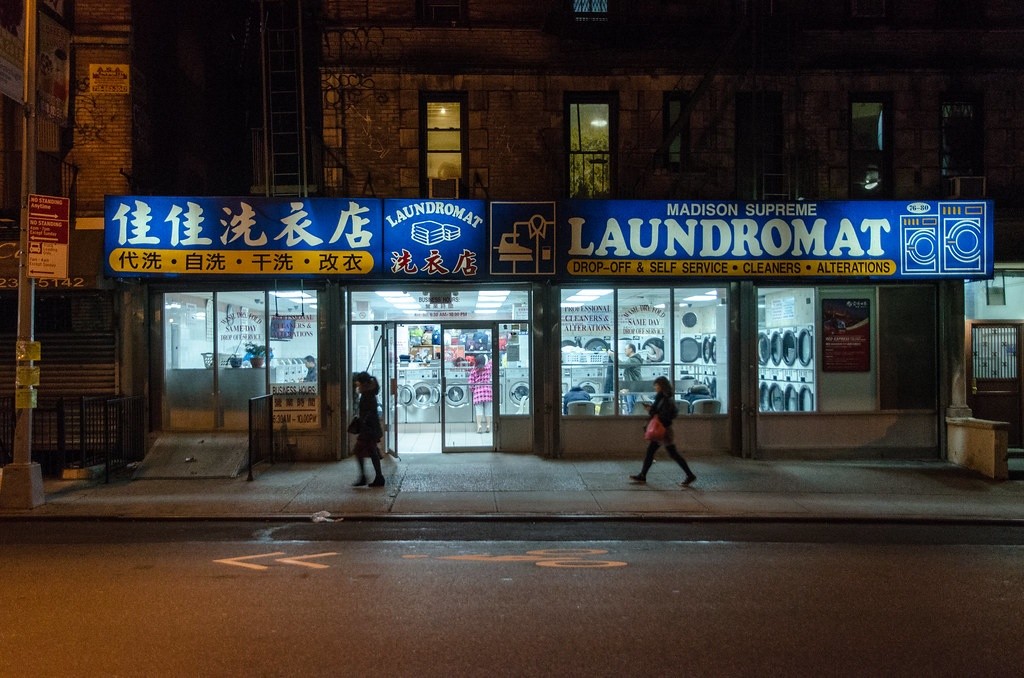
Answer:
270, 317, 295, 339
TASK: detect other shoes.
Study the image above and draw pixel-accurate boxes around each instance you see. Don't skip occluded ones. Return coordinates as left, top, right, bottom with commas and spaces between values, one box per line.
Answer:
629, 473, 647, 482
369, 475, 385, 487
354, 478, 367, 486
486, 426, 490, 433
478, 425, 482, 433
681, 474, 696, 484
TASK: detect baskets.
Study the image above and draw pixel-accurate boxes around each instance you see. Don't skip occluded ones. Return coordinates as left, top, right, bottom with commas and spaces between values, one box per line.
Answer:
201, 353, 232, 370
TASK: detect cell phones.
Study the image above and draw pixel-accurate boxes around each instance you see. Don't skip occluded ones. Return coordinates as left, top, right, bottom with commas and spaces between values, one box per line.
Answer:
643, 403, 646, 407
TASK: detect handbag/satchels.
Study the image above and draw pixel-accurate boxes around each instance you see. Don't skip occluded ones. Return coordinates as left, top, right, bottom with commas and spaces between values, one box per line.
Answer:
346, 416, 361, 434
643, 415, 665, 441
411, 327, 440, 362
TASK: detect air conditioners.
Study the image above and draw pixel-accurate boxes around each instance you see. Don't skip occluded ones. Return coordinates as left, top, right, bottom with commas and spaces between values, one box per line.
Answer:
429, 177, 461, 200
940, 176, 987, 199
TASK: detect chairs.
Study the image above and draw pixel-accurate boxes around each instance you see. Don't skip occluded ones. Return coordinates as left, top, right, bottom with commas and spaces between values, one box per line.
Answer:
600, 401, 622, 416
567, 400, 595, 415
675, 399, 691, 415
631, 401, 653, 416
691, 399, 721, 415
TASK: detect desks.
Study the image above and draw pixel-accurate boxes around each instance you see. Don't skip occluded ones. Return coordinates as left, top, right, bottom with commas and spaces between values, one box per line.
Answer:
561, 392, 686, 401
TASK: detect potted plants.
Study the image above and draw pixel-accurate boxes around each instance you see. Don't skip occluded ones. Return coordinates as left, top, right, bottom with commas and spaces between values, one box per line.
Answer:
246, 341, 272, 368
227, 354, 242, 369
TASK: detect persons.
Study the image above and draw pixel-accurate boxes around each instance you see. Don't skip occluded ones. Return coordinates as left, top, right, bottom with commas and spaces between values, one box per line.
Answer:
469, 355, 492, 433
608, 344, 642, 414
352, 371, 385, 488
564, 386, 590, 415
300, 355, 316, 382
629, 376, 696, 487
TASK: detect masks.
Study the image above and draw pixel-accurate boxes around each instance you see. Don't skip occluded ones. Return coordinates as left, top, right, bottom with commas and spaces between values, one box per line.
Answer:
356, 387, 360, 395
